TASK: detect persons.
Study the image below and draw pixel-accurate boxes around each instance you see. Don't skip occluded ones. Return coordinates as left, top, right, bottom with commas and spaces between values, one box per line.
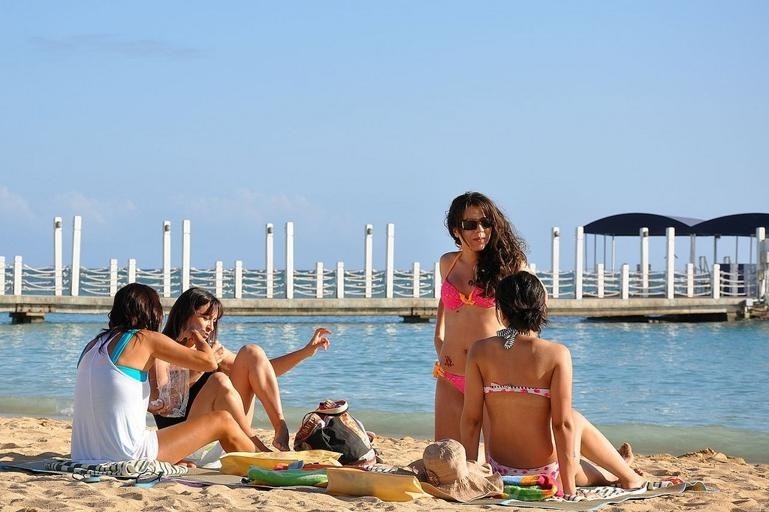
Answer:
147, 288, 332, 451
69, 283, 261, 465
461, 272, 648, 496
432, 192, 547, 442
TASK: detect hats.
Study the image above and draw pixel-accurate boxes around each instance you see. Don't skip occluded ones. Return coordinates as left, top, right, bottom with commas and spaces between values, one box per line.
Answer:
400, 437, 504, 503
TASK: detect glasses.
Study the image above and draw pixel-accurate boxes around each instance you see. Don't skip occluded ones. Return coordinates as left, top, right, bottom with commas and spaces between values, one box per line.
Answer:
462, 218, 493, 230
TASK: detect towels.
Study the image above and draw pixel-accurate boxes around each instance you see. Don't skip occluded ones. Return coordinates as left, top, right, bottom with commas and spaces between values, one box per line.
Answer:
543, 479, 650, 503
42, 458, 190, 480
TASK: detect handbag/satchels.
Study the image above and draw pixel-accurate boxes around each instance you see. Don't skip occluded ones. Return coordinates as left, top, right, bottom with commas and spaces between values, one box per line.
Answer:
295, 399, 376, 465
